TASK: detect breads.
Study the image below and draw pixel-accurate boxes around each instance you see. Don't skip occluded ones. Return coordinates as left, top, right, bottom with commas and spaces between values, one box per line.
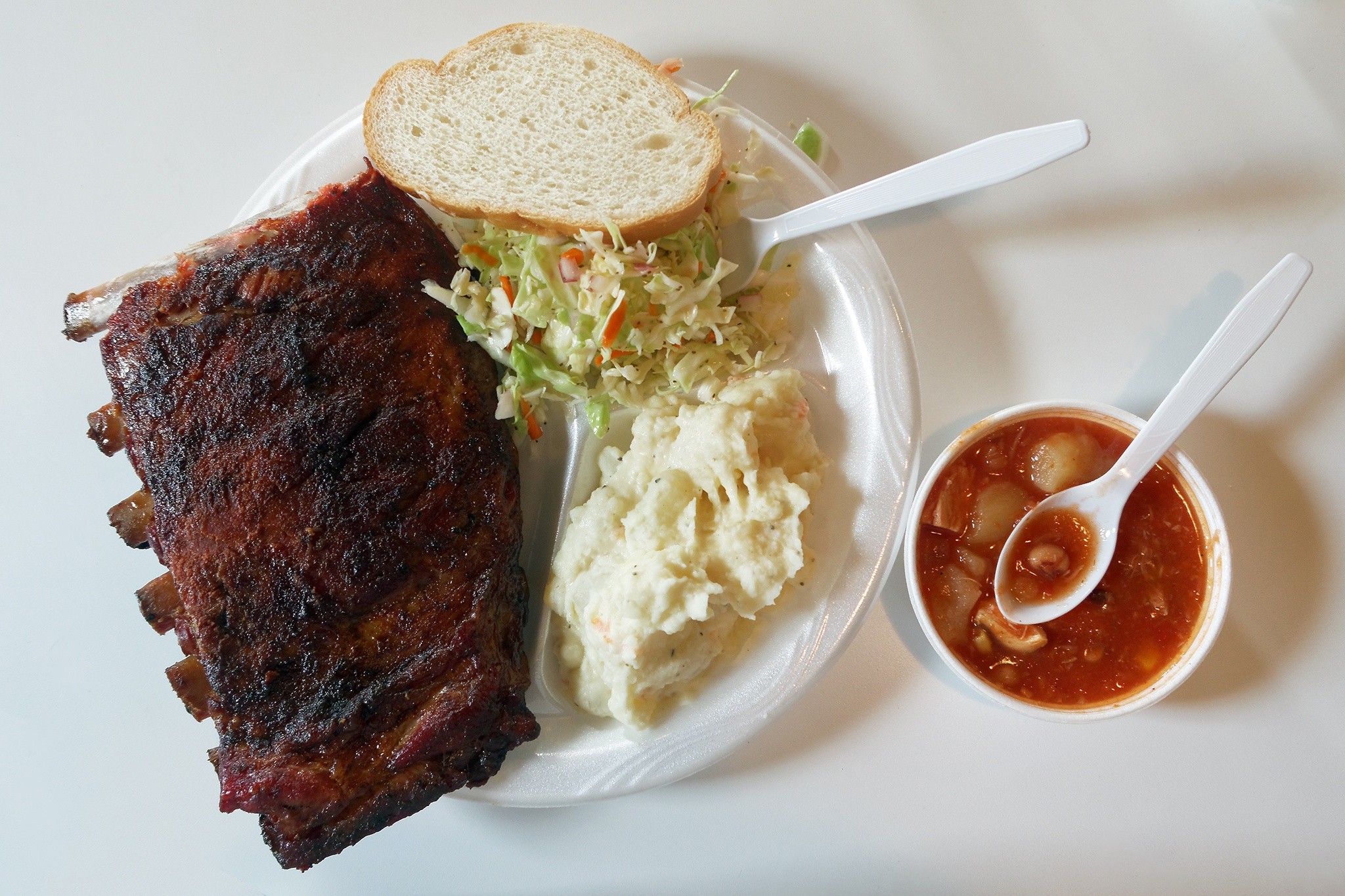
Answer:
356, 24, 732, 253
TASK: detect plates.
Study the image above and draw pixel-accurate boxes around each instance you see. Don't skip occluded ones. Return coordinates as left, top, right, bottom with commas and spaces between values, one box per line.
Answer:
236, 74, 921, 813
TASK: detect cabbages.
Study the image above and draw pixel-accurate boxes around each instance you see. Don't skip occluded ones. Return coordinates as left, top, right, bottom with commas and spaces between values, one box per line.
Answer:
408, 60, 801, 436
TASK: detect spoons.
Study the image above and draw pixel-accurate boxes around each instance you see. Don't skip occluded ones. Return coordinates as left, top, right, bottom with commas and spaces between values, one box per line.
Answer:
724, 116, 1090, 309
995, 253, 1313, 625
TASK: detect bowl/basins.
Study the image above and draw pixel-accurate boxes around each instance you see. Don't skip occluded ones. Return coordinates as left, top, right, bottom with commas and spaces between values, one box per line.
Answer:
902, 400, 1233, 721
